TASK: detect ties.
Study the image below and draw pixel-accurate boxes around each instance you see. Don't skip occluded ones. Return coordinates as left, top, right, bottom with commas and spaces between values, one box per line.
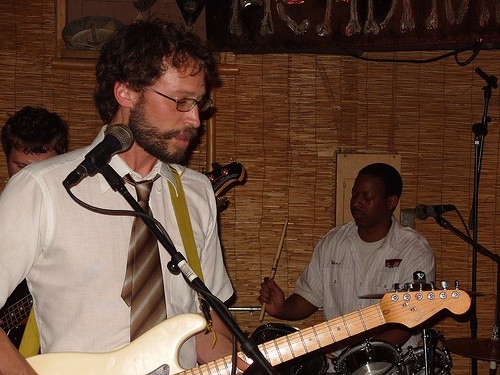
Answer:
120, 174, 168, 343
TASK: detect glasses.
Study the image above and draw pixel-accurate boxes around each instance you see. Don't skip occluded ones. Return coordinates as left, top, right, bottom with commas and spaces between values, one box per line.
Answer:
137, 82, 214, 113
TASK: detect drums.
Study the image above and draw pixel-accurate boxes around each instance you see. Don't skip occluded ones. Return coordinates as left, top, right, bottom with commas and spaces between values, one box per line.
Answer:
244, 322, 329, 375
335, 337, 402, 375
401, 346, 451, 375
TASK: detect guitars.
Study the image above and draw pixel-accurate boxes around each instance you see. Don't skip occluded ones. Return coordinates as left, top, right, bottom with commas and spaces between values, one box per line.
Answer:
0, 161, 247, 331
24, 279, 470, 375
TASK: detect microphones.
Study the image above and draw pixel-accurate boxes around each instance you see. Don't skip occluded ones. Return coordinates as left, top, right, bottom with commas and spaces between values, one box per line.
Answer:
64, 124, 134, 187
415, 204, 455, 219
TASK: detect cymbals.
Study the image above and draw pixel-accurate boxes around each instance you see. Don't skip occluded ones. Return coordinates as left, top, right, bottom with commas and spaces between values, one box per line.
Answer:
443, 337, 500, 362
356, 290, 491, 295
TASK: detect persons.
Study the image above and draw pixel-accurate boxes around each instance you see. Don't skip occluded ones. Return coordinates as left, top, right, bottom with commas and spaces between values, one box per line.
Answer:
258, 163, 437, 374
0, 23, 236, 375
0, 105, 71, 359
